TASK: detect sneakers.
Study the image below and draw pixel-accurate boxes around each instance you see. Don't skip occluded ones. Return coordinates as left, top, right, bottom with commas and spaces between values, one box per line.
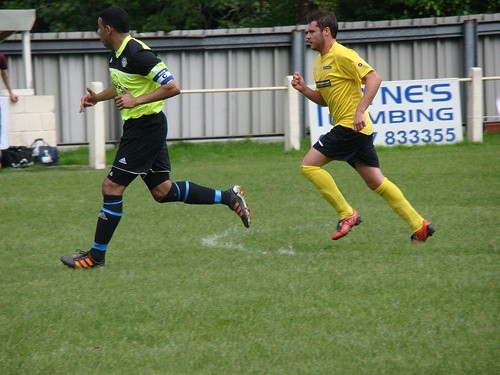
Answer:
228, 185, 251, 229
410, 219, 437, 244
331, 208, 363, 240
60, 248, 106, 270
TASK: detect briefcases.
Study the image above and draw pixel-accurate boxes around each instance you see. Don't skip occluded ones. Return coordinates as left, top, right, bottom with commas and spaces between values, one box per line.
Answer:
0, 138, 58, 168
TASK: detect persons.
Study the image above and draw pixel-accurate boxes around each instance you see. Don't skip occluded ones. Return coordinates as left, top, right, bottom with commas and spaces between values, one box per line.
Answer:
291, 11, 438, 245
60, 7, 251, 269
0, 52, 18, 103
